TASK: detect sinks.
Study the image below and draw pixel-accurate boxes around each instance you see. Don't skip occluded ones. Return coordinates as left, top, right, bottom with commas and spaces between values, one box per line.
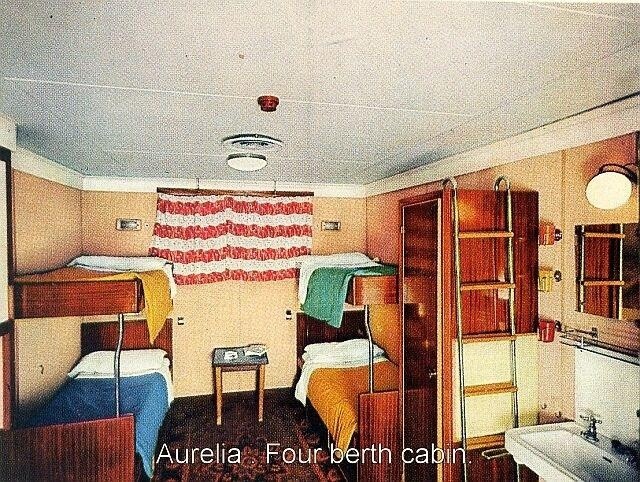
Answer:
505, 419, 637, 482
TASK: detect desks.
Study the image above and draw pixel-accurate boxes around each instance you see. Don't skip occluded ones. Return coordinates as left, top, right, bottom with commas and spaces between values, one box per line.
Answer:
213, 347, 270, 429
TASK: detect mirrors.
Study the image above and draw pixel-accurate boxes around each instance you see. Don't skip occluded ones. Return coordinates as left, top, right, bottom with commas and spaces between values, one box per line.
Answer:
573, 221, 640, 325
398, 190, 442, 482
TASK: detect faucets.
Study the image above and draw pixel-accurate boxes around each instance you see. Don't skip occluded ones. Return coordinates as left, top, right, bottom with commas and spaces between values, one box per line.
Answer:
579, 414, 602, 441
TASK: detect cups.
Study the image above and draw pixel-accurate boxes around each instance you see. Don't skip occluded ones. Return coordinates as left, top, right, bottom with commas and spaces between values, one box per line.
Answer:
538, 267, 552, 292
539, 222, 554, 245
539, 319, 554, 342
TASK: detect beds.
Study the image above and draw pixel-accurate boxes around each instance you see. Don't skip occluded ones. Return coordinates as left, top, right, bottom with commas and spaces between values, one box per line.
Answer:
295, 252, 400, 481
13, 253, 178, 480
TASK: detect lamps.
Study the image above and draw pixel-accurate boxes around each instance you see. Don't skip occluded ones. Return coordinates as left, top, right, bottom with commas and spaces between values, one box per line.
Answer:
585, 156, 640, 210
226, 154, 268, 172
